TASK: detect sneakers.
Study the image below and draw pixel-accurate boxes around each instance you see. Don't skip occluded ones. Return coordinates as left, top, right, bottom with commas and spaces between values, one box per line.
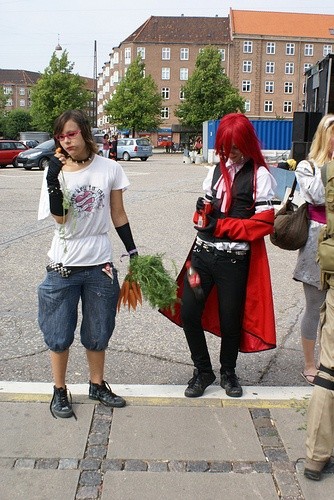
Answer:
50, 385, 77, 421
185, 367, 216, 397
89, 380, 126, 408
220, 368, 242, 397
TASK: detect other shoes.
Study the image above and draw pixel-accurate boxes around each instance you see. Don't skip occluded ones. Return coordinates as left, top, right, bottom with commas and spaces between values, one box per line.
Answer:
304, 455, 329, 481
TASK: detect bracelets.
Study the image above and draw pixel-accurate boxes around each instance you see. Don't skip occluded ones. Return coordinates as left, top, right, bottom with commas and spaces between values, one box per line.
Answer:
129, 249, 138, 255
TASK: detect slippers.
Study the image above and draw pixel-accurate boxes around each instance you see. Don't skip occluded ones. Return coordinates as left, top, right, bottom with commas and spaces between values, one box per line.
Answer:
301, 372, 315, 386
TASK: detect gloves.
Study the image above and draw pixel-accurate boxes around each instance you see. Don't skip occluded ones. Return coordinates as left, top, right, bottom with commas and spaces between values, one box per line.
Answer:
194, 215, 217, 234
196, 197, 205, 215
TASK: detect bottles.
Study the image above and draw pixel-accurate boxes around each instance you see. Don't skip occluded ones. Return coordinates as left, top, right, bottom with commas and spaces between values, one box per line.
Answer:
193, 193, 213, 227
185, 261, 203, 299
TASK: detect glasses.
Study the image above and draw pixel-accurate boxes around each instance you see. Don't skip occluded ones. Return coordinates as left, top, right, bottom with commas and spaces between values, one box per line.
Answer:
54, 129, 82, 141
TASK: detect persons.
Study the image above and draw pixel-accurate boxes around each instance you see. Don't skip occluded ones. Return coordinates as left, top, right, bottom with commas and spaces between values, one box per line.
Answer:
36, 109, 139, 420
196, 137, 201, 154
102, 134, 117, 161
181, 113, 278, 398
292, 115, 334, 481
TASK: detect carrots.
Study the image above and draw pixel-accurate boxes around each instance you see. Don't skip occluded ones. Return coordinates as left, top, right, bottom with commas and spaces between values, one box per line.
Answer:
117, 280, 143, 313
55, 147, 61, 153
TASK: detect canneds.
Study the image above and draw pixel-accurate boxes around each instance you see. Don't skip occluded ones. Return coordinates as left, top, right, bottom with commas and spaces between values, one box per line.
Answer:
198, 200, 210, 228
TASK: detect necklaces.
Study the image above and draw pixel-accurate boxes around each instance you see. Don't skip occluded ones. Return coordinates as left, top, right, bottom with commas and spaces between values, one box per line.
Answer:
68, 150, 94, 165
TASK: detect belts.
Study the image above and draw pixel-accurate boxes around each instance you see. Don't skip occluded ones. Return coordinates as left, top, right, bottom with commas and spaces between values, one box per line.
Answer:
45, 256, 107, 278
196, 236, 250, 255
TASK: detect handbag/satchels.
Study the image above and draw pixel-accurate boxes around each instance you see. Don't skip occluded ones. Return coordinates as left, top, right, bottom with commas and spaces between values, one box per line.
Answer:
270, 160, 315, 250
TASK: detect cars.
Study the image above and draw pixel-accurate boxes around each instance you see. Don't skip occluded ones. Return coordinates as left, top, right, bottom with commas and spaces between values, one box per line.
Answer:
93, 134, 105, 147
16, 139, 39, 148
97, 137, 153, 161
0, 139, 29, 168
16, 139, 56, 170
